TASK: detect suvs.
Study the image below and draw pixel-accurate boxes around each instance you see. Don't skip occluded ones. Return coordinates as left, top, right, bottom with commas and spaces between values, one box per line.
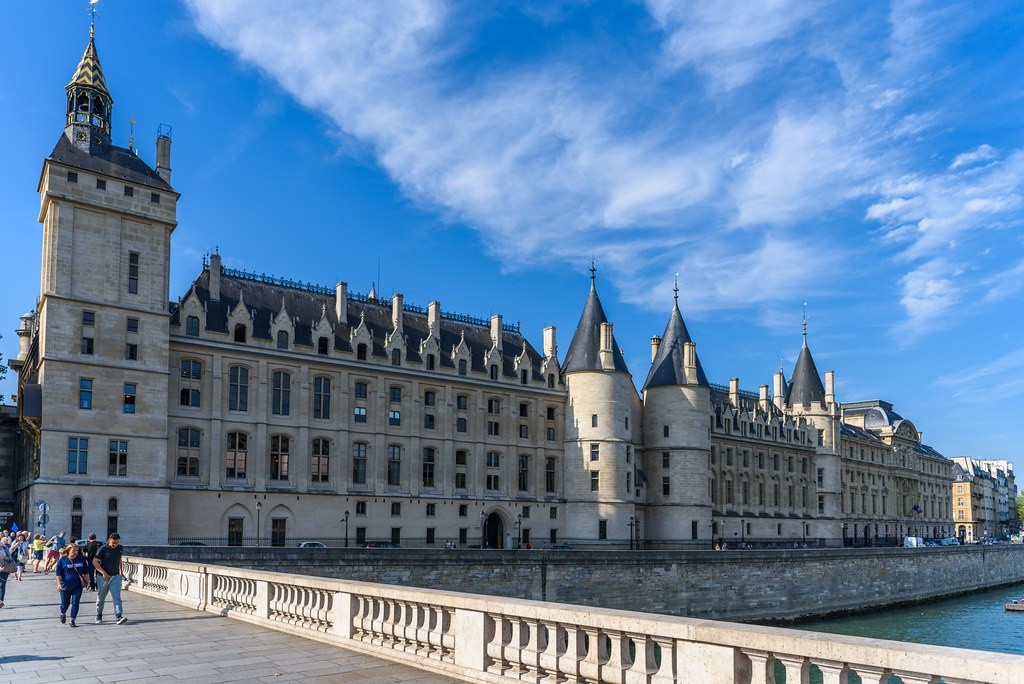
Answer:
361, 540, 402, 548
296, 541, 326, 548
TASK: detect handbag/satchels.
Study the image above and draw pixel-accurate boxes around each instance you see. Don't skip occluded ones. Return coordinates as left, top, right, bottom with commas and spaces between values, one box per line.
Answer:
18, 554, 28, 563
81, 577, 87, 587
0, 558, 17, 574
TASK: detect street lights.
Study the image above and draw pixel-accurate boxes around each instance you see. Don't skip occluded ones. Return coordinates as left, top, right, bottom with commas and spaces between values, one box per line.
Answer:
256, 500, 263, 546
740, 518, 745, 542
344, 510, 350, 547
629, 516, 634, 549
482, 512, 489, 548
801, 521, 806, 544
517, 513, 523, 549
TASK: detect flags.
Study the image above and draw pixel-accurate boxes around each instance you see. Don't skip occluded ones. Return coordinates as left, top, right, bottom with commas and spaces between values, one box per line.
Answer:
913, 505, 922, 514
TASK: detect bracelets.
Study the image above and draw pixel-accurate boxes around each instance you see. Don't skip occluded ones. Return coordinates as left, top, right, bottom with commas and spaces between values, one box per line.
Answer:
58, 582, 61, 585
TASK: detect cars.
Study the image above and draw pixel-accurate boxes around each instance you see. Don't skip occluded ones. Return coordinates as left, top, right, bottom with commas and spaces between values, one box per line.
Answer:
75, 540, 106, 547
178, 541, 208, 546
923, 536, 998, 546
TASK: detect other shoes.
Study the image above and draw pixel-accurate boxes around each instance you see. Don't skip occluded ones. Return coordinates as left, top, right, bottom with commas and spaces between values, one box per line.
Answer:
34, 570, 41, 573
15, 574, 18, 580
18, 577, 21, 581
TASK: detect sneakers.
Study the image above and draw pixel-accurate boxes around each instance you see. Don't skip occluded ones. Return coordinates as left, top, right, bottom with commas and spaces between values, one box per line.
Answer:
95, 614, 102, 623
69, 620, 78, 627
60, 614, 66, 624
88, 589, 95, 592
116, 617, 128, 625
0, 601, 5, 608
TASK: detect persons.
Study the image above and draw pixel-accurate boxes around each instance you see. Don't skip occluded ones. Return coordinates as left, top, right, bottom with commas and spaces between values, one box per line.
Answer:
635, 537, 640, 550
445, 540, 455, 548
68, 532, 106, 591
989, 536, 993, 542
33, 534, 46, 573
748, 543, 753, 549
794, 541, 798, 549
55, 543, 91, 627
802, 542, 807, 548
44, 531, 66, 570
526, 542, 546, 549
92, 532, 128, 624
714, 543, 727, 550
0, 529, 34, 607
983, 536, 986, 542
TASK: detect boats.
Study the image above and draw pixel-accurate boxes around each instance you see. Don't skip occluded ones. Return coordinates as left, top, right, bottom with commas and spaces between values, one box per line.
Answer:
1003, 599, 1024, 611
